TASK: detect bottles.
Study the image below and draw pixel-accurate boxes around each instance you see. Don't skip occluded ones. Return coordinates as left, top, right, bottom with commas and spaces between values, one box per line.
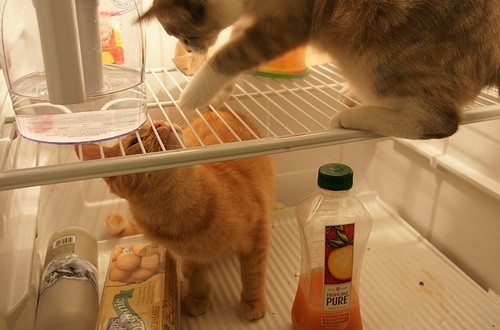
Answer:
34, 225, 100, 329
98, 9, 127, 67
291, 162, 373, 330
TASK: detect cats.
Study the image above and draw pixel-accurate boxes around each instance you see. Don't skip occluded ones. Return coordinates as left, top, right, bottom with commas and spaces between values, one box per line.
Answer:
132, 0, 500, 140
74, 108, 276, 321
